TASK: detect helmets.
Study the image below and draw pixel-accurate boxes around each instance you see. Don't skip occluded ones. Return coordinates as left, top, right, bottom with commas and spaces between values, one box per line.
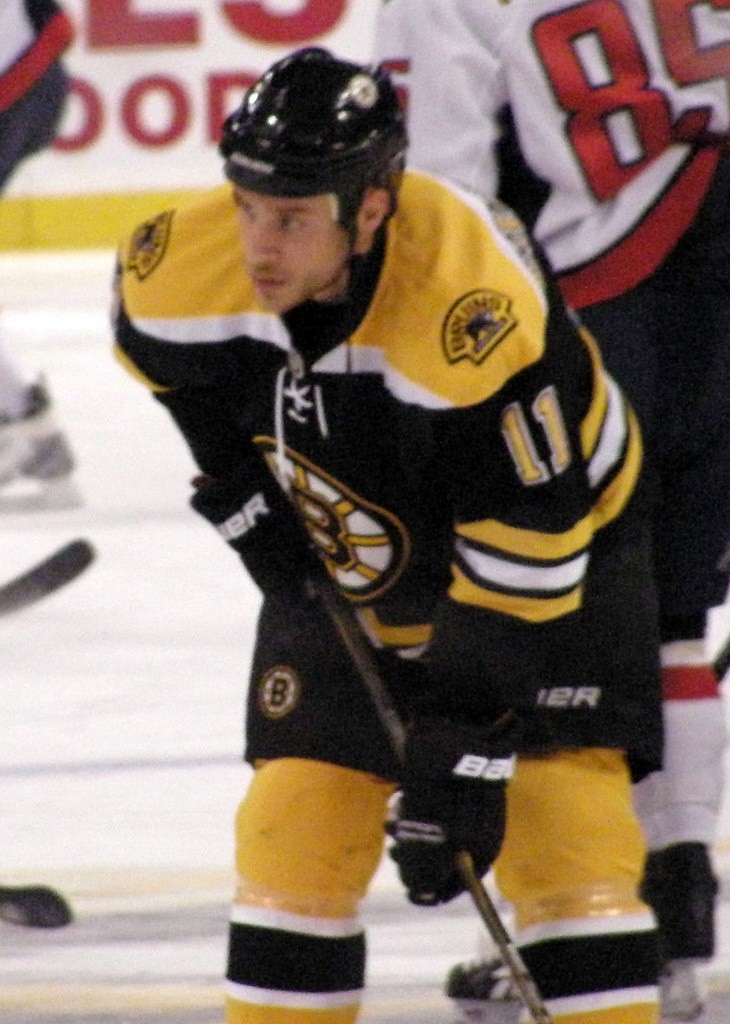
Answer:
220, 44, 410, 198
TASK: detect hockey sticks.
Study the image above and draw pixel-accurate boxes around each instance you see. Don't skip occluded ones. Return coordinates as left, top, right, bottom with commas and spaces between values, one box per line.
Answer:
0, 537, 96, 618
0, 884, 74, 928
301, 547, 553, 1024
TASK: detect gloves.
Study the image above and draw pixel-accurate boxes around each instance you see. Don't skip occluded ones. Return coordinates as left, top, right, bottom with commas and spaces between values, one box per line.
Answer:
382, 702, 525, 909
185, 454, 328, 614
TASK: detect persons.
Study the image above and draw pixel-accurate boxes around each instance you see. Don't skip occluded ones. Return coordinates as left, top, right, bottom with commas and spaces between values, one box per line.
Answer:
373, 0, 730, 1024
107, 46, 669, 1023
1, 0, 78, 485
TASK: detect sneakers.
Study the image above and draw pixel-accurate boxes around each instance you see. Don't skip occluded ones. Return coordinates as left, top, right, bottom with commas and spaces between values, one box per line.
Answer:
0, 377, 82, 512
641, 838, 722, 1020
